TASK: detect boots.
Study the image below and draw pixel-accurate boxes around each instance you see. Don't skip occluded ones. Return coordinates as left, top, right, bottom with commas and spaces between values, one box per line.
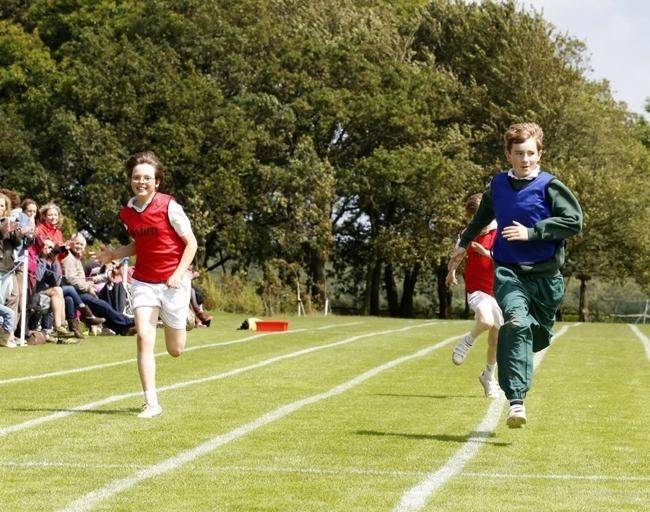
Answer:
79, 305, 107, 329
69, 319, 85, 339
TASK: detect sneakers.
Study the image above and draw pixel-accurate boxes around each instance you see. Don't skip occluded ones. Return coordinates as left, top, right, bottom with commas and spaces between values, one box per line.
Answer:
137, 405, 162, 419
506, 405, 527, 430
0, 325, 81, 348
479, 369, 500, 398
452, 333, 473, 365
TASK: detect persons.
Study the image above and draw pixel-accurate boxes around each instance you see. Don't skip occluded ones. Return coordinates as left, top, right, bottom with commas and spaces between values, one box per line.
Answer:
448, 191, 505, 399
89, 150, 200, 421
448, 121, 584, 429
1, 188, 214, 350
